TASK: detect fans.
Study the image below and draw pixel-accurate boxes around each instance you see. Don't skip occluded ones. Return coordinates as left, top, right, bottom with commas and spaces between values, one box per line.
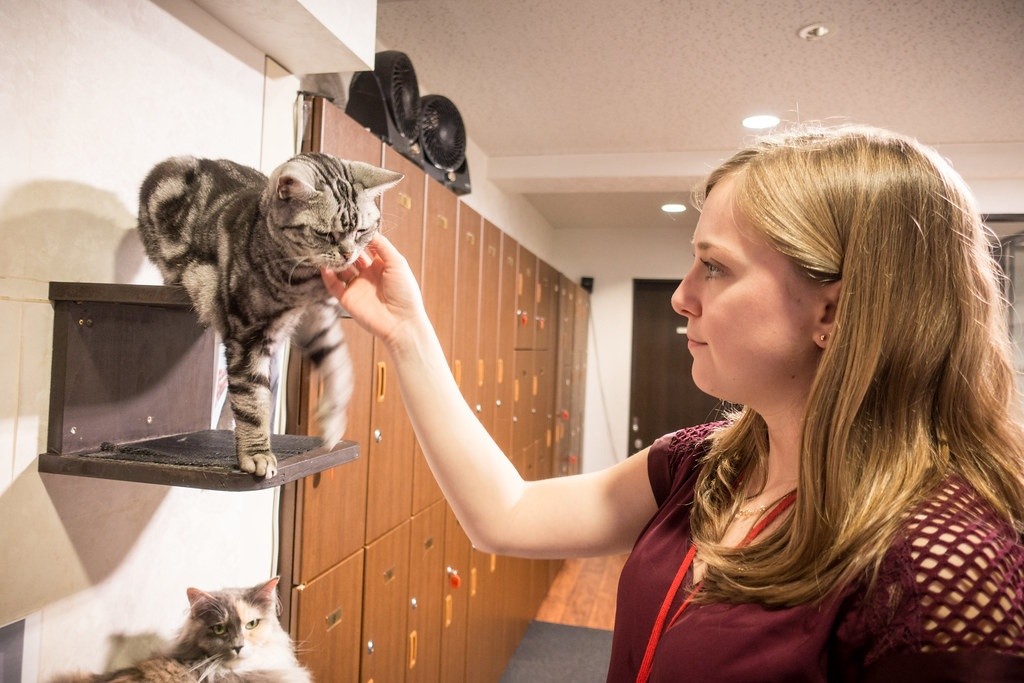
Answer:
344, 49, 473, 197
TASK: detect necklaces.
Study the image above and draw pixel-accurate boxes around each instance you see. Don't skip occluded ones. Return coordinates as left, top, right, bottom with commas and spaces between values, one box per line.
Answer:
734, 506, 765, 519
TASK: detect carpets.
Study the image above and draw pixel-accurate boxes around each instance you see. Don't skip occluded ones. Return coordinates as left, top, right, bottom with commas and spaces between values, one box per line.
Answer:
499, 620, 615, 683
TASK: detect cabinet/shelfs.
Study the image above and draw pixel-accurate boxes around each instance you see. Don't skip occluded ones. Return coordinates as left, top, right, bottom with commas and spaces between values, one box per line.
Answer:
36, 279, 363, 494
275, 91, 591, 683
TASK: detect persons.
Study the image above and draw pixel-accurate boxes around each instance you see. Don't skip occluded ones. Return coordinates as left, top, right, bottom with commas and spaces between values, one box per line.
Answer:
321, 126, 1023, 682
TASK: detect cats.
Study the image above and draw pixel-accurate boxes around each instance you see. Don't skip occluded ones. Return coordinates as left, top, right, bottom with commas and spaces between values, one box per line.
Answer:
52, 576, 312, 683
138, 152, 407, 479
